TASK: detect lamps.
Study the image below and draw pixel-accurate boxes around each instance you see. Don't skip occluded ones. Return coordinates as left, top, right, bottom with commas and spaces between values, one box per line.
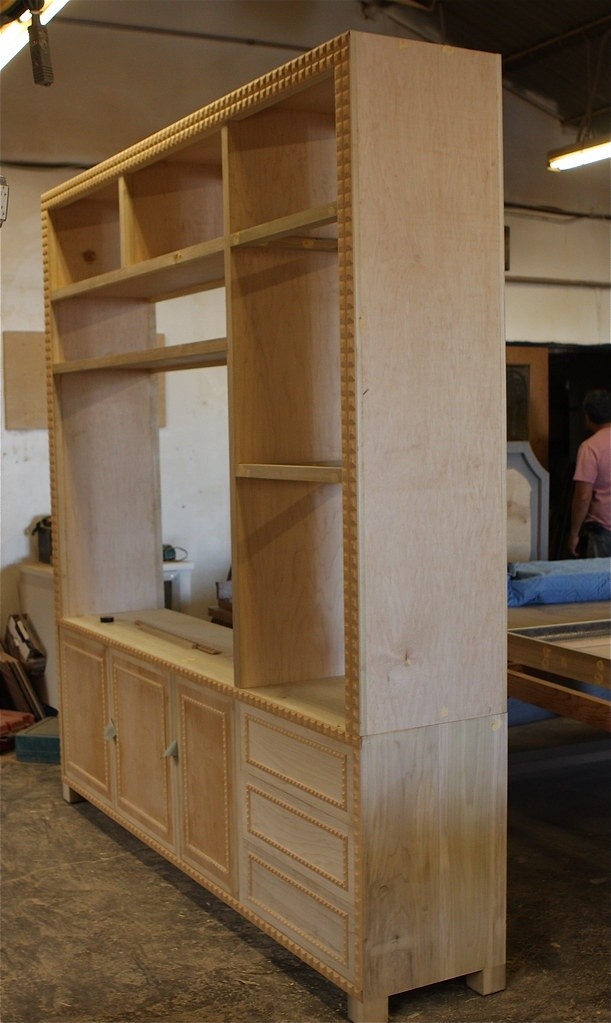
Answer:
544, 132, 611, 173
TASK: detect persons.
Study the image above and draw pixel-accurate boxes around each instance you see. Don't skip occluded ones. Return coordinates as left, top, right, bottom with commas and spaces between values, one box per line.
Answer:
566, 392, 611, 559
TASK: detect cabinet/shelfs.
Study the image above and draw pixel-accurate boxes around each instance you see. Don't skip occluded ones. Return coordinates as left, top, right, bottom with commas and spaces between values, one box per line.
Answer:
18, 560, 195, 711
39, 31, 505, 1023
